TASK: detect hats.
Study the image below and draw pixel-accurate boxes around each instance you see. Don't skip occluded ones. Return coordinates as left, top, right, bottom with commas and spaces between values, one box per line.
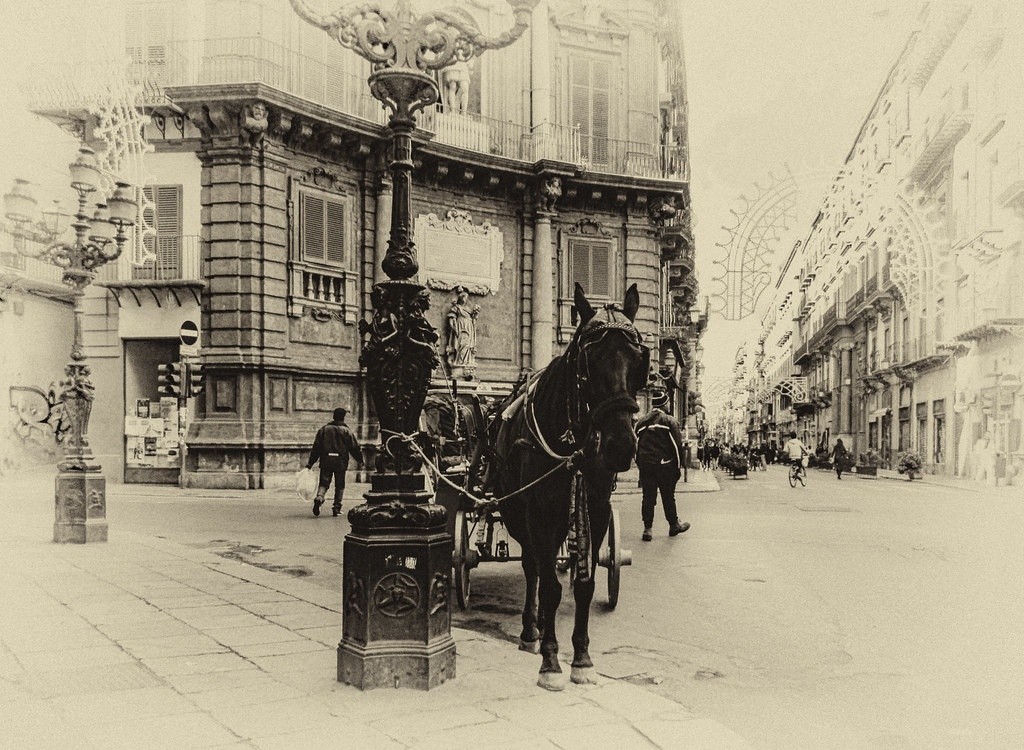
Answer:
651, 391, 669, 406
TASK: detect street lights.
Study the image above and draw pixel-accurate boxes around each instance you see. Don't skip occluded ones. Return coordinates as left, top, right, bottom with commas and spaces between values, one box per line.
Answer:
287, 0, 538, 697
50, 144, 147, 548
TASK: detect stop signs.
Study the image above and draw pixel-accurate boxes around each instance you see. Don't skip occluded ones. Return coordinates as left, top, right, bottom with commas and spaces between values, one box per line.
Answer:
180, 320, 199, 346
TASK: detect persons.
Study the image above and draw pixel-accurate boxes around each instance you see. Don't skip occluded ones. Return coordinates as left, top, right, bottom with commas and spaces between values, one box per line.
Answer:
633, 392, 690, 541
703, 441, 719, 471
305, 407, 365, 518
831, 438, 848, 480
445, 293, 481, 381
783, 431, 808, 481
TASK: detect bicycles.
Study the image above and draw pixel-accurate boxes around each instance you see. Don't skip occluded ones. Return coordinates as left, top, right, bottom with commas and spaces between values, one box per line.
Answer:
788, 459, 808, 487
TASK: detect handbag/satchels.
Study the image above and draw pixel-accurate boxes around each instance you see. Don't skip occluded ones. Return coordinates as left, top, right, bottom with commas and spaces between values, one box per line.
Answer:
829, 454, 835, 464
295, 467, 319, 503
802, 457, 809, 467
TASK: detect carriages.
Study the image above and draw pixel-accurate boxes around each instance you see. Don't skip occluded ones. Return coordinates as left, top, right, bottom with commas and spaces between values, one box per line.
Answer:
423, 280, 653, 693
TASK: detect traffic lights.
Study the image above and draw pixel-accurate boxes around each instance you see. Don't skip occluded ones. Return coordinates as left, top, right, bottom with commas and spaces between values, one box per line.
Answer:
167, 361, 186, 399
183, 361, 204, 397
157, 364, 166, 394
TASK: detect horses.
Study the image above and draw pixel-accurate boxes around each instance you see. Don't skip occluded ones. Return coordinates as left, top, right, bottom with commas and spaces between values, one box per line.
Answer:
489, 280, 651, 693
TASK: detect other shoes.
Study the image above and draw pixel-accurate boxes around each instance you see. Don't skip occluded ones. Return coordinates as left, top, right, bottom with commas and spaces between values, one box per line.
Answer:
716, 467, 718, 469
669, 522, 690, 536
643, 528, 652, 541
333, 511, 344, 517
313, 498, 322, 516
